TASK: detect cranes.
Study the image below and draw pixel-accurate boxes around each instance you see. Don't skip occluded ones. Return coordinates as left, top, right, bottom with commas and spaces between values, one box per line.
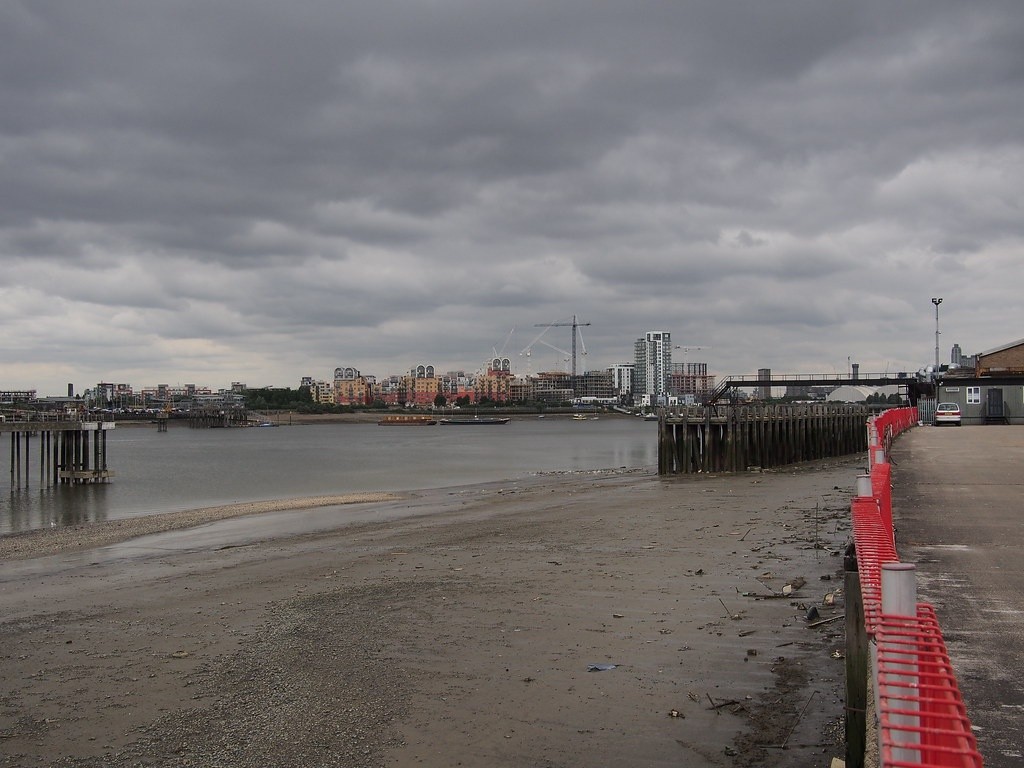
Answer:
672, 345, 713, 375
534, 314, 591, 389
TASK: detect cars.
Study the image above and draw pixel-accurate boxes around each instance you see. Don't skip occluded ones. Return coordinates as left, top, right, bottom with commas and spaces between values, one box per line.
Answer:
934, 402, 961, 426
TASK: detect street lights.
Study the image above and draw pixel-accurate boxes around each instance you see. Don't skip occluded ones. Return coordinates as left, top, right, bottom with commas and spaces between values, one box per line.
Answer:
932, 297, 943, 411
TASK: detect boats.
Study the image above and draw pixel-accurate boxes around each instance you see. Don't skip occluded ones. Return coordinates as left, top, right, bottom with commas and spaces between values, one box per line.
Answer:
377, 415, 437, 426
573, 414, 587, 420
539, 416, 545, 418
644, 412, 658, 421
257, 421, 276, 427
439, 417, 510, 426
